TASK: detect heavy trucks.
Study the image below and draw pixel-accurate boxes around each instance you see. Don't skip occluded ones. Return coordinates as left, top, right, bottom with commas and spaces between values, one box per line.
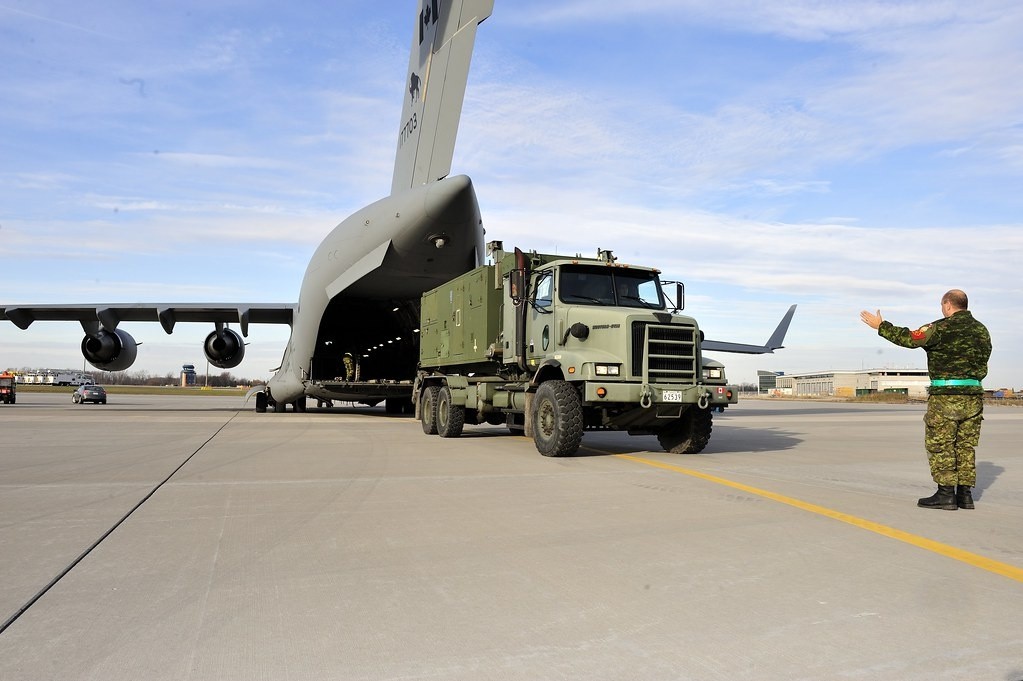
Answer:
700, 356, 739, 413
417, 242, 738, 460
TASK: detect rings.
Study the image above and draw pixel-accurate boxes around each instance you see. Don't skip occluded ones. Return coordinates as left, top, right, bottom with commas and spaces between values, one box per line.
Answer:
866, 319, 868, 321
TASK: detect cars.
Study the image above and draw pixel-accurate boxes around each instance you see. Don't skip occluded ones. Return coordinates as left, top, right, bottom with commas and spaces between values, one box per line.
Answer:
71, 385, 107, 404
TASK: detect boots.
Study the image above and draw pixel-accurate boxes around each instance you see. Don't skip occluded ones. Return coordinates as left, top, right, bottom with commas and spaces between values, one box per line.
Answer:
918, 484, 958, 510
954, 484, 975, 509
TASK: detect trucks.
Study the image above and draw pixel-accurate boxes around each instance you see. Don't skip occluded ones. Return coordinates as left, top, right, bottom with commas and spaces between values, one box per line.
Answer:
0, 371, 17, 404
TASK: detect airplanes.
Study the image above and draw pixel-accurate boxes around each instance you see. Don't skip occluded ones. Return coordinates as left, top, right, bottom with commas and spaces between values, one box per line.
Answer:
0, 0, 798, 415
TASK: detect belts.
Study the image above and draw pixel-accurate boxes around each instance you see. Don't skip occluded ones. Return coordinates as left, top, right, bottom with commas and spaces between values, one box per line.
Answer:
930, 378, 982, 387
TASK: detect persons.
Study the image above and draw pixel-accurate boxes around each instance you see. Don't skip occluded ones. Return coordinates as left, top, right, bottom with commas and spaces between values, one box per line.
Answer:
343, 352, 354, 381
617, 282, 628, 299
860, 289, 992, 510
